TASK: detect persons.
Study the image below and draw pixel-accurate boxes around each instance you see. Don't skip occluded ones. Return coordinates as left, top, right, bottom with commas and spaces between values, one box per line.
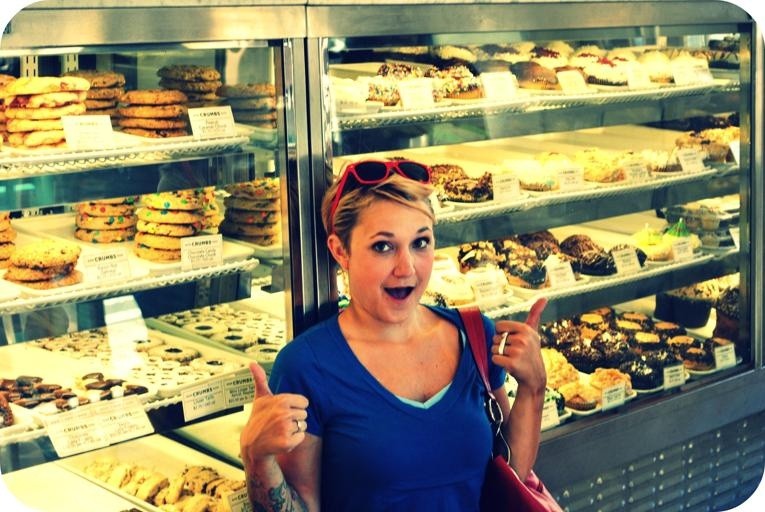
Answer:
239, 158, 548, 512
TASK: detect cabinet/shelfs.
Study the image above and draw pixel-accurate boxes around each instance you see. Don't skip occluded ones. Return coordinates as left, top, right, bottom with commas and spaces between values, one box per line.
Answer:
307, 0, 765, 512
0, 0, 317, 512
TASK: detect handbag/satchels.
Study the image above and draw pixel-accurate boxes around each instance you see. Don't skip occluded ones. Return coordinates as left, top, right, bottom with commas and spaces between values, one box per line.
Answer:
457, 304, 566, 512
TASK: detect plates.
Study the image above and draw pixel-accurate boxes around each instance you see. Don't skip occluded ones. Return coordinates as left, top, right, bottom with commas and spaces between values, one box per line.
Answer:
549, 226, 702, 270
502, 141, 646, 197
1, 346, 157, 414
576, 263, 649, 285
451, 247, 591, 298
616, 299, 719, 345
162, 303, 294, 367
56, 433, 247, 512
519, 76, 599, 101
535, 368, 636, 416
632, 371, 690, 396
180, 413, 250, 467
710, 67, 739, 77
332, 77, 454, 110
49, 326, 249, 401
3, 465, 149, 512
1, 117, 135, 157
115, 108, 249, 143
335, 269, 513, 322
333, 61, 530, 110
247, 289, 290, 320
3, 235, 149, 297
609, 308, 742, 375
539, 389, 572, 429
384, 149, 517, 215
587, 80, 661, 95
333, 163, 455, 224
323, 80, 380, 114
239, 112, 278, 142
223, 225, 288, 260
676, 201, 741, 220
559, 134, 709, 182
520, 178, 592, 194
1, 284, 21, 308
13, 211, 254, 274
648, 76, 730, 93
596, 214, 739, 255
1, 406, 36, 439
604, 126, 736, 175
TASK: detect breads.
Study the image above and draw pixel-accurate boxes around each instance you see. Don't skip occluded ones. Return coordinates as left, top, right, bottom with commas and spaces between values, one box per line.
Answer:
578, 49, 713, 89
683, 347, 711, 371
561, 236, 616, 274
367, 76, 402, 107
8, 93, 77, 108
425, 268, 474, 305
76, 229, 133, 244
11, 242, 81, 267
459, 238, 548, 288
429, 165, 494, 203
620, 311, 653, 328
76, 204, 134, 218
629, 225, 671, 260
505, 151, 572, 191
3, 75, 89, 94
118, 104, 188, 119
134, 245, 180, 261
139, 209, 204, 224
186, 92, 218, 100
158, 79, 222, 93
0, 213, 15, 268
633, 332, 665, 354
671, 336, 701, 361
616, 320, 649, 337
221, 97, 275, 110
589, 306, 614, 320
591, 331, 634, 367
665, 217, 701, 251
563, 343, 602, 374
589, 368, 632, 394
119, 118, 186, 129
377, 64, 441, 81
618, 361, 659, 387
644, 350, 679, 380
4, 267, 74, 280
517, 230, 576, 277
573, 312, 610, 330
648, 149, 682, 172
576, 148, 626, 181
87, 89, 124, 99
85, 110, 116, 118
90, 194, 137, 205
226, 178, 278, 198
83, 459, 246, 512
124, 129, 188, 138
218, 221, 276, 238
0, 291, 286, 426
157, 65, 221, 81
216, 83, 275, 97
122, 90, 186, 105
135, 223, 196, 237
134, 233, 183, 250
704, 337, 738, 362
143, 189, 215, 210
432, 42, 605, 71
6, 119, 63, 131
241, 235, 276, 247
5, 107, 82, 119
84, 100, 115, 109
222, 195, 279, 213
511, 61, 564, 91
233, 111, 277, 121
8, 133, 63, 146
15, 271, 84, 288
675, 131, 728, 165
433, 65, 485, 102
75, 217, 135, 231
654, 323, 685, 344
540, 318, 582, 349
610, 245, 646, 268
245, 121, 277, 128
63, 70, 125, 87
541, 348, 578, 389
224, 209, 278, 225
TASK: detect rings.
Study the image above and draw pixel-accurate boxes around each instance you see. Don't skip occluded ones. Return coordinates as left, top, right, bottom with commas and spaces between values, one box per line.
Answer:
293, 419, 302, 432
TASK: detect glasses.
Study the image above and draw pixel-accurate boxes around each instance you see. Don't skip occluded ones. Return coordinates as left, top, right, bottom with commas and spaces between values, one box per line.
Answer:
330, 159, 432, 238
